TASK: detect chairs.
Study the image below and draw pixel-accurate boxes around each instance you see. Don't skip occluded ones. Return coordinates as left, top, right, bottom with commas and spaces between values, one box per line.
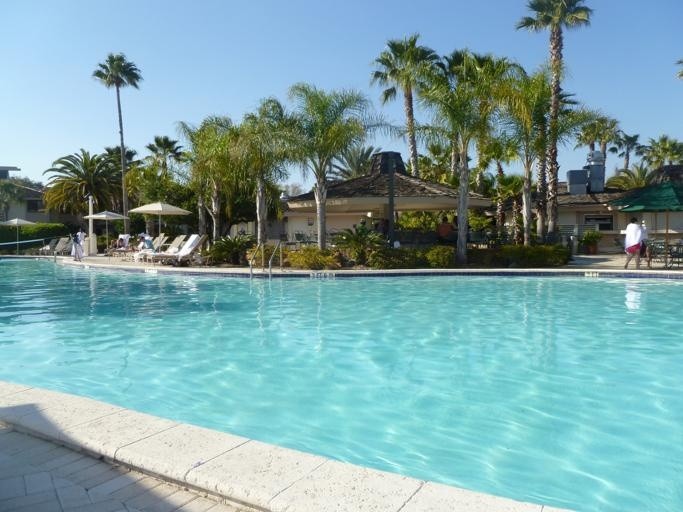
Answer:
108, 232, 209, 266
41, 236, 73, 257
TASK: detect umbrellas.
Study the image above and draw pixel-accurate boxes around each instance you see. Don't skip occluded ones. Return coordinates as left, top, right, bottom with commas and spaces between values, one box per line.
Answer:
0, 217, 36, 254
127, 201, 192, 252
610, 181, 683, 255
82, 209, 132, 247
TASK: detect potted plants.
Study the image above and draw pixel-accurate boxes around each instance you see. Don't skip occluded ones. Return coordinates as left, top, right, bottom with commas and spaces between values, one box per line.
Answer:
582, 228, 605, 253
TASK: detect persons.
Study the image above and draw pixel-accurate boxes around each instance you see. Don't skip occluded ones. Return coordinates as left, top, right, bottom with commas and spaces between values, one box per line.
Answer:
625, 283, 642, 312
69, 228, 88, 261
623, 217, 651, 269
128, 235, 153, 253
438, 216, 458, 239
111, 238, 123, 248
